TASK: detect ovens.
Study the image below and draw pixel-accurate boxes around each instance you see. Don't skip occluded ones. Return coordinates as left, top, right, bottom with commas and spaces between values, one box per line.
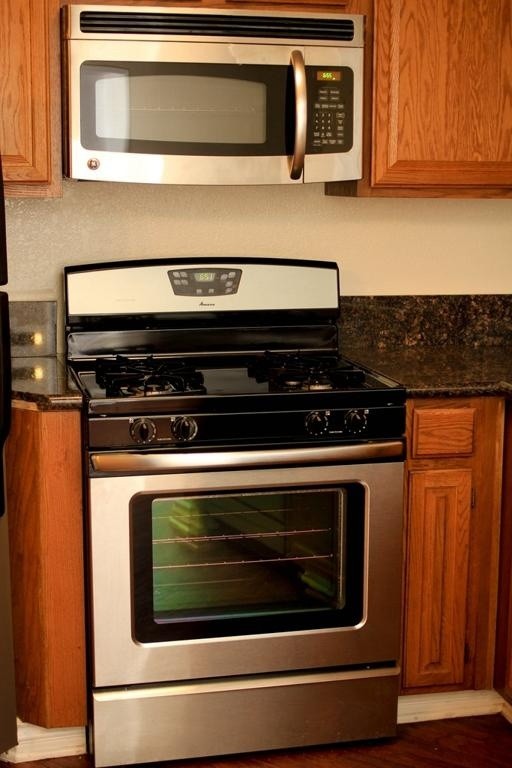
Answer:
86, 448, 402, 764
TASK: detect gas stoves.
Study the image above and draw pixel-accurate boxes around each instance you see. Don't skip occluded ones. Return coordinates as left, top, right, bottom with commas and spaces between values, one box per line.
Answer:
67, 348, 406, 449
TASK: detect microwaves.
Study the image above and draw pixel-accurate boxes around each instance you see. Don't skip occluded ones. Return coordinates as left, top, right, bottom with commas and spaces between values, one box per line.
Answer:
61, 3, 364, 185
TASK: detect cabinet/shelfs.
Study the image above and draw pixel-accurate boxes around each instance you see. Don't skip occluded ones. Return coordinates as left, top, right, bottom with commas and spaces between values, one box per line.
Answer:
324, 1, 511, 199
